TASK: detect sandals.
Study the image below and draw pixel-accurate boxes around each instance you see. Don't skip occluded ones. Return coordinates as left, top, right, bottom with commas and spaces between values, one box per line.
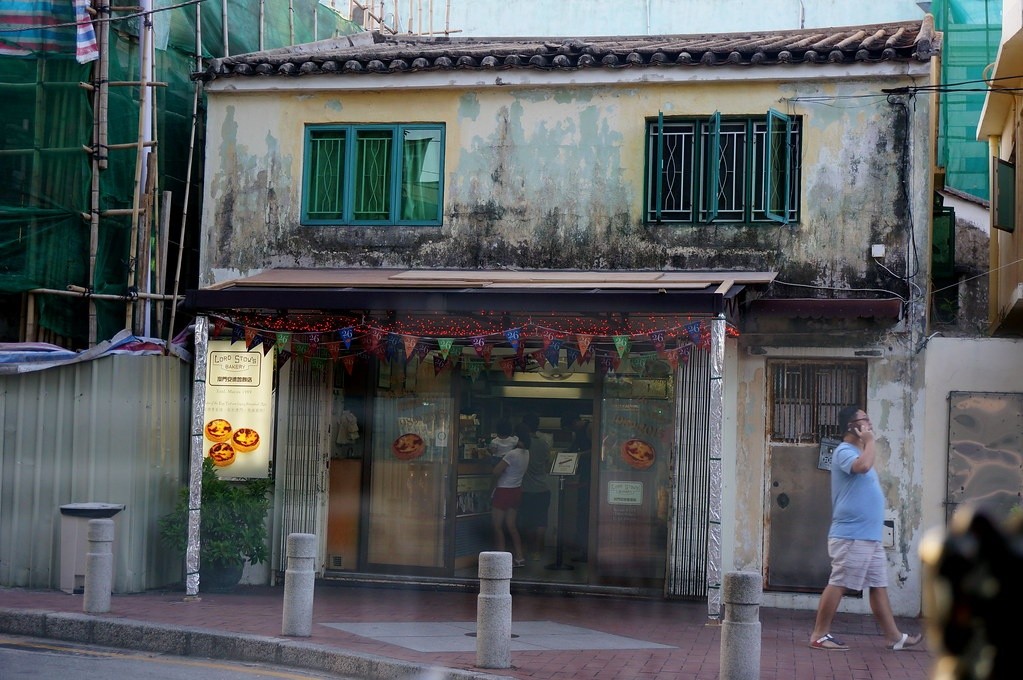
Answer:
512, 559, 526, 567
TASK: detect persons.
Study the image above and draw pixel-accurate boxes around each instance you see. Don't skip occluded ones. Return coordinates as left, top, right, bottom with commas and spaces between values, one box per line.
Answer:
807, 405, 924, 649
477, 411, 592, 567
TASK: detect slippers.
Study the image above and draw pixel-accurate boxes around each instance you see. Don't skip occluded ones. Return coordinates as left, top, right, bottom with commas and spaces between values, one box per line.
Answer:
886, 632, 921, 650
808, 632, 851, 651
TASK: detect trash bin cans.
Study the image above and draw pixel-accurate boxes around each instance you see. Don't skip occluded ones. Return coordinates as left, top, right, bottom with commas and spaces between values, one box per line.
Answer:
57, 502, 125, 595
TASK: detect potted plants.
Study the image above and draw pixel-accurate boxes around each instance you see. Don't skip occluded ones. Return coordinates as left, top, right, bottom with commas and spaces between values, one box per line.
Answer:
157, 456, 274, 594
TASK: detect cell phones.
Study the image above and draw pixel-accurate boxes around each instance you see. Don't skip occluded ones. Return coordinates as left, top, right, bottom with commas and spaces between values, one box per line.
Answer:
846, 423, 862, 438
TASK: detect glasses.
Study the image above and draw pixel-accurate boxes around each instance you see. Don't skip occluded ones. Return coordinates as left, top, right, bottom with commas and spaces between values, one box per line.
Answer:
851, 415, 869, 422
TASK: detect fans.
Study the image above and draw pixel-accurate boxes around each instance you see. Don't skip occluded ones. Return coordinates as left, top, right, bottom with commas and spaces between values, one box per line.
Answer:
538, 360, 575, 381
